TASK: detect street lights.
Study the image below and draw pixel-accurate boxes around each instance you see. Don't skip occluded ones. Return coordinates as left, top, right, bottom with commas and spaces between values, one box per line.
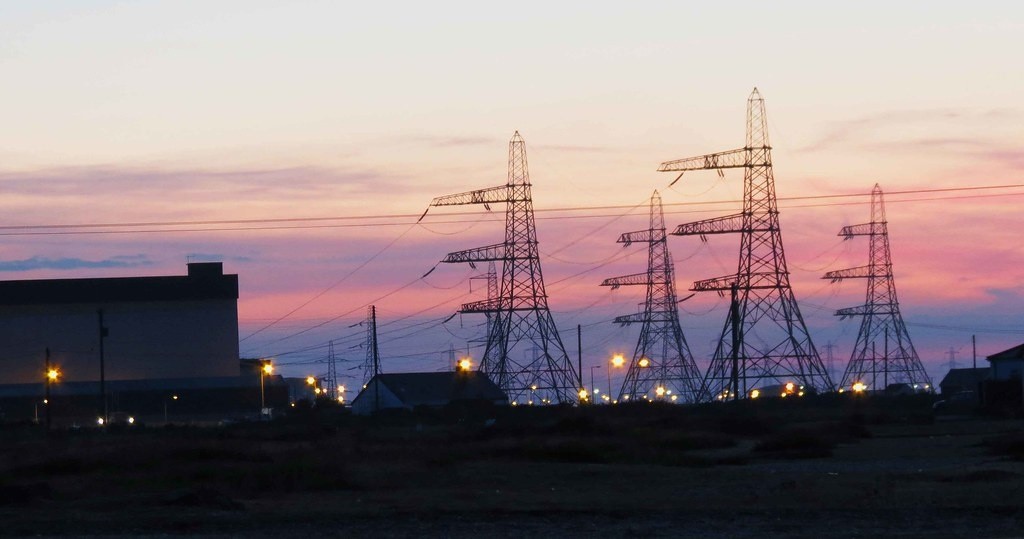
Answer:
607, 356, 622, 404
45, 368, 57, 436
590, 365, 601, 405
260, 364, 271, 408
164, 394, 177, 422
